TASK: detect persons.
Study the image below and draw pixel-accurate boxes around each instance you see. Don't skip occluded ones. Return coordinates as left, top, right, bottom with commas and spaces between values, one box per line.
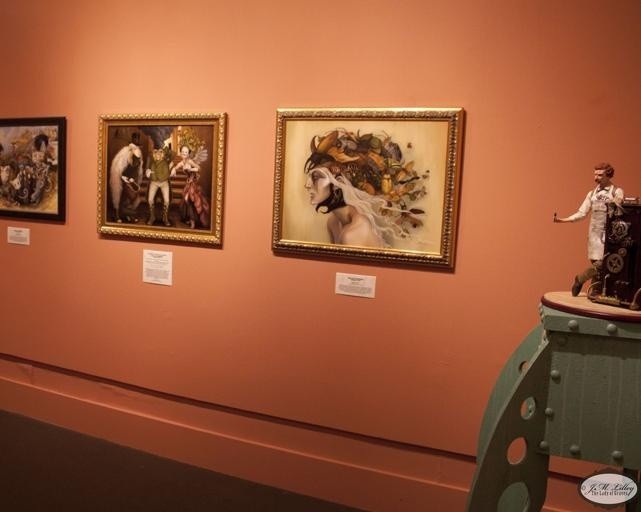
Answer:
108, 143, 213, 230
303, 128, 429, 249
552, 162, 625, 297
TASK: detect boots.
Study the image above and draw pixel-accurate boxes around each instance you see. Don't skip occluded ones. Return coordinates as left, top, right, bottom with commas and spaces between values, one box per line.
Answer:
147, 204, 171, 226
113, 208, 123, 223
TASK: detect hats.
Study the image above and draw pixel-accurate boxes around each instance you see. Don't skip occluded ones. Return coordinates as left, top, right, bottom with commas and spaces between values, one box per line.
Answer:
129, 133, 143, 146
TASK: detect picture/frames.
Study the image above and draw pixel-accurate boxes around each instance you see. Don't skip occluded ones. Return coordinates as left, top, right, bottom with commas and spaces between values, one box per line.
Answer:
0, 117, 68, 222
97, 111, 226, 247
268, 107, 467, 273
